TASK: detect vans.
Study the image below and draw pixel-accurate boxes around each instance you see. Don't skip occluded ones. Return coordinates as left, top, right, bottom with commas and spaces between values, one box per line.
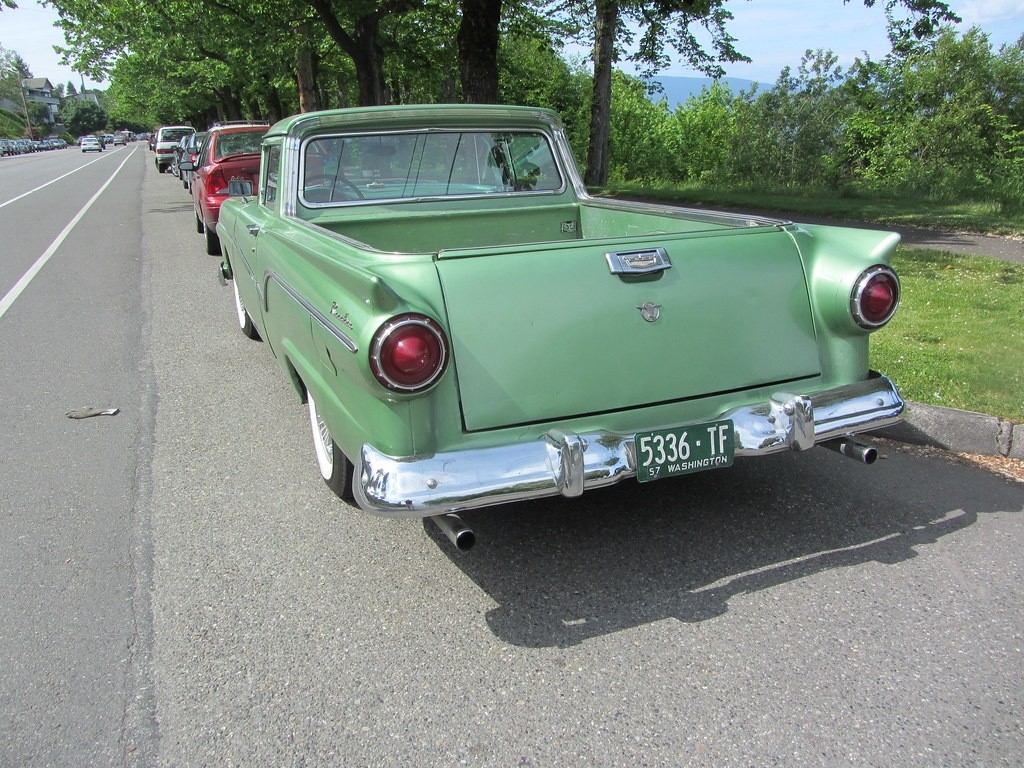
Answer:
155, 127, 196, 173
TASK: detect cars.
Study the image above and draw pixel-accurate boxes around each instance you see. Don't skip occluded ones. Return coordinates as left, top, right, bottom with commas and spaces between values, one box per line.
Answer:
171, 122, 345, 255
78, 135, 114, 146
130, 133, 147, 142
114, 135, 126, 146
0, 139, 67, 156
150, 133, 158, 151
81, 138, 102, 152
96, 138, 105, 149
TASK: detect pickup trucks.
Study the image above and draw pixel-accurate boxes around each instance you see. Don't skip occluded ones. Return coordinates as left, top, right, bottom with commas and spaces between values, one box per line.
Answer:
216, 104, 908, 553
